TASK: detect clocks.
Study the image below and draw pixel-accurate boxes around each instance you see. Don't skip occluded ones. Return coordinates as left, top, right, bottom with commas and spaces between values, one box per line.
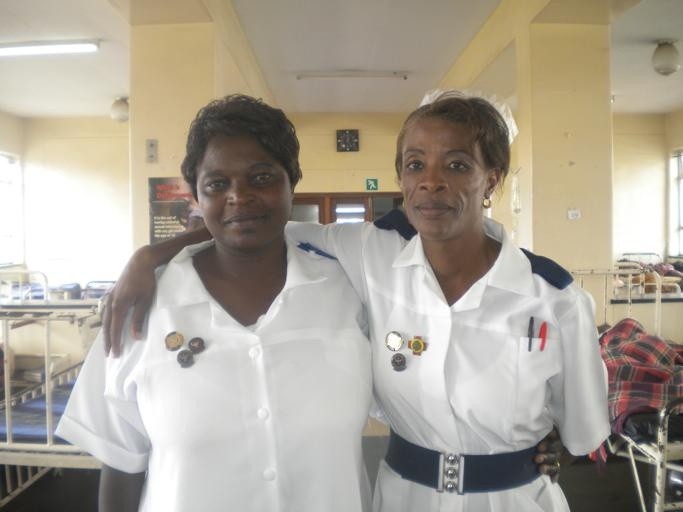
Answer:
336, 128, 359, 152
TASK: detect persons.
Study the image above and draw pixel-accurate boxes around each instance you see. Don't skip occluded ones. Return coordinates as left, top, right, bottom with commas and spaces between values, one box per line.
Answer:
169, 196, 205, 234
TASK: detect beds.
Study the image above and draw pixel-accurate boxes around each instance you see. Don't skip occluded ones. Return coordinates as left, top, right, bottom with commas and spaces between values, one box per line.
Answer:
570, 266, 683, 512
0, 267, 120, 510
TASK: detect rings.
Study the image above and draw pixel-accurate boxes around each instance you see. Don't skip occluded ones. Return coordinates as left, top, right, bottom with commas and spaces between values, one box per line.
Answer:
554, 460, 560, 469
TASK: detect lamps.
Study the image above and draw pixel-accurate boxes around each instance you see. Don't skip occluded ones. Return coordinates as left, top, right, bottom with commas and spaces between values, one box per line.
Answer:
652, 38, 681, 76
109, 97, 129, 122
0, 38, 101, 56
